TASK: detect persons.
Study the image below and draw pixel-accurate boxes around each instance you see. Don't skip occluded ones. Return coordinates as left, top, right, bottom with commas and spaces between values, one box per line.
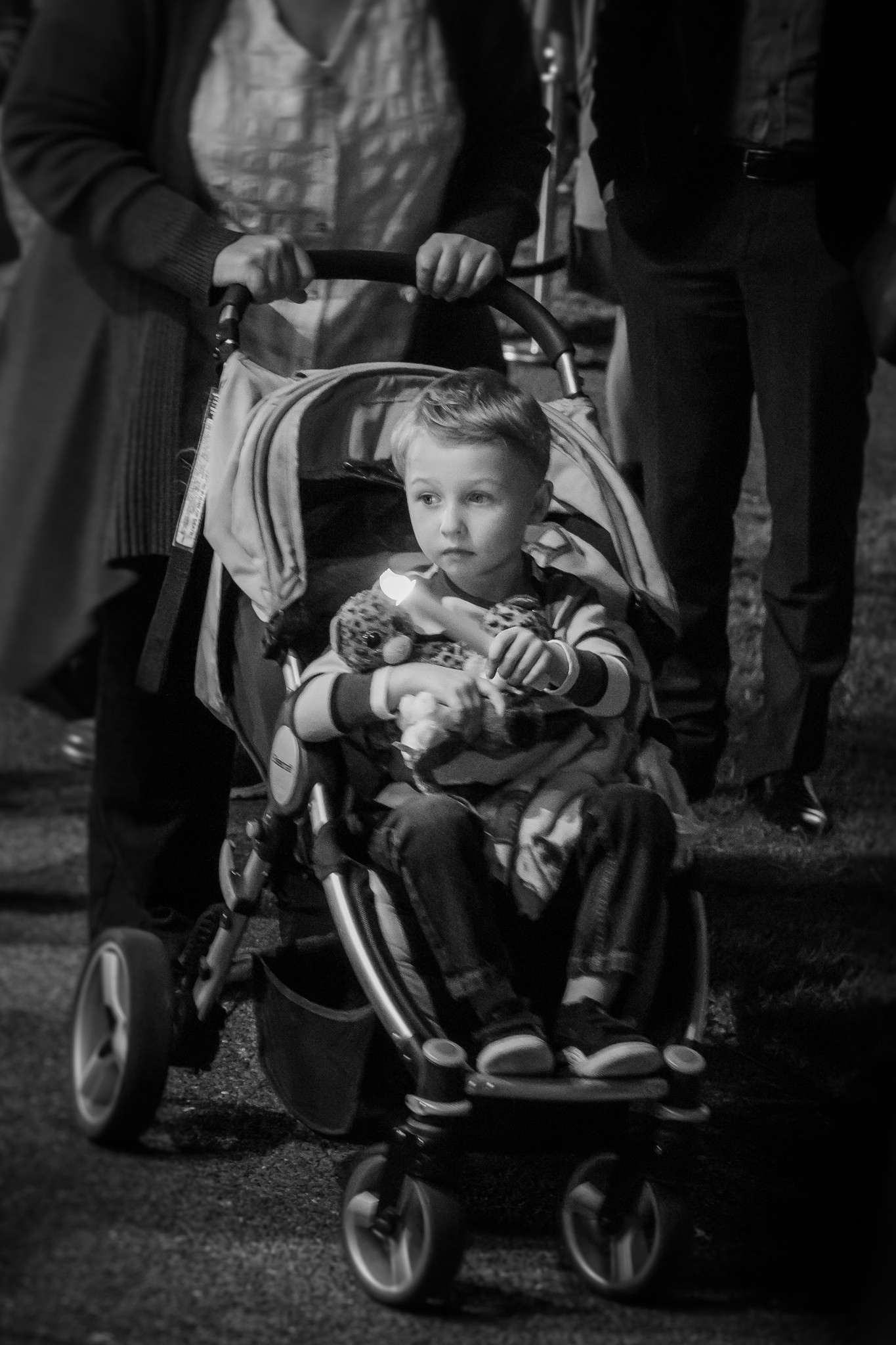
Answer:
277, 366, 677, 1080
1, 0, 896, 1028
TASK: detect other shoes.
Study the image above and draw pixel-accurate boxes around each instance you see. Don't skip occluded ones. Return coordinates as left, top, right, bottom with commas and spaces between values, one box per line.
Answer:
554, 999, 660, 1077
63, 720, 95, 762
477, 1024, 553, 1075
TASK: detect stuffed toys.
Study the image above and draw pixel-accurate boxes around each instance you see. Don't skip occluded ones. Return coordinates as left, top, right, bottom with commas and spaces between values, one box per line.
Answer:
331, 577, 554, 750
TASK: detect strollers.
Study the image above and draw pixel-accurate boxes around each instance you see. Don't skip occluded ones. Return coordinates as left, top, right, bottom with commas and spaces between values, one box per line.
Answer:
70, 250, 711, 1310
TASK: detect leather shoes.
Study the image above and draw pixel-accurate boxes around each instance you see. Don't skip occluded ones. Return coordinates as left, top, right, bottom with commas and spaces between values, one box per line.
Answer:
684, 766, 717, 803
748, 770, 826, 836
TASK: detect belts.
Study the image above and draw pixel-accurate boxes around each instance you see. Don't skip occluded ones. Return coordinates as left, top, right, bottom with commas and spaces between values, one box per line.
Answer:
727, 148, 816, 179
